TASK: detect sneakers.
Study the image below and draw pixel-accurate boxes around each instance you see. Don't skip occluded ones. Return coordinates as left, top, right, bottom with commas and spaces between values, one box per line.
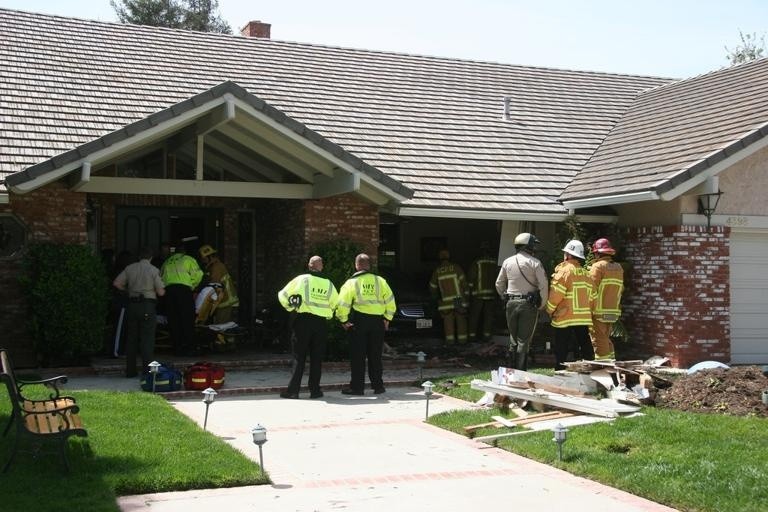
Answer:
374, 387, 385, 394
310, 392, 323, 399
280, 392, 298, 398
342, 388, 363, 394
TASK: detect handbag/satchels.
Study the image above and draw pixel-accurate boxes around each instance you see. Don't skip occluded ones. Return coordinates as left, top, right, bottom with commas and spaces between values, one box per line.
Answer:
184, 362, 224, 390
141, 361, 182, 392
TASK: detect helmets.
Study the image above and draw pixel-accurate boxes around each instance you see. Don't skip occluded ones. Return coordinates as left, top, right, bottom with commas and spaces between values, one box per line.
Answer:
199, 246, 215, 256
561, 240, 585, 260
593, 239, 616, 253
514, 233, 540, 245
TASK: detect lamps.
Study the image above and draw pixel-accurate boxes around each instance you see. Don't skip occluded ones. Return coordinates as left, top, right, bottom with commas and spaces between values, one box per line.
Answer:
148, 361, 161, 394
552, 422, 570, 461
202, 388, 217, 431
416, 352, 427, 379
250, 423, 268, 474
698, 176, 724, 232
421, 381, 436, 420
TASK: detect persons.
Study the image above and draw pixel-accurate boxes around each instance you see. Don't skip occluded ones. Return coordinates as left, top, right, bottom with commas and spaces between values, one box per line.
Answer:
112, 246, 166, 378
544, 238, 594, 371
334, 253, 397, 395
587, 237, 624, 361
467, 239, 501, 343
101, 236, 241, 357
427, 249, 469, 346
495, 232, 549, 372
277, 255, 339, 398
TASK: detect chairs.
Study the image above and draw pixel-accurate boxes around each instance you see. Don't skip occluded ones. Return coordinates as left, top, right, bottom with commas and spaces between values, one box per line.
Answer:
0, 349, 87, 474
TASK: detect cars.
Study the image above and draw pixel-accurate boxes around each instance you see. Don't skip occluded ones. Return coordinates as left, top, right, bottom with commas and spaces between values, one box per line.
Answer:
386, 296, 442, 337
